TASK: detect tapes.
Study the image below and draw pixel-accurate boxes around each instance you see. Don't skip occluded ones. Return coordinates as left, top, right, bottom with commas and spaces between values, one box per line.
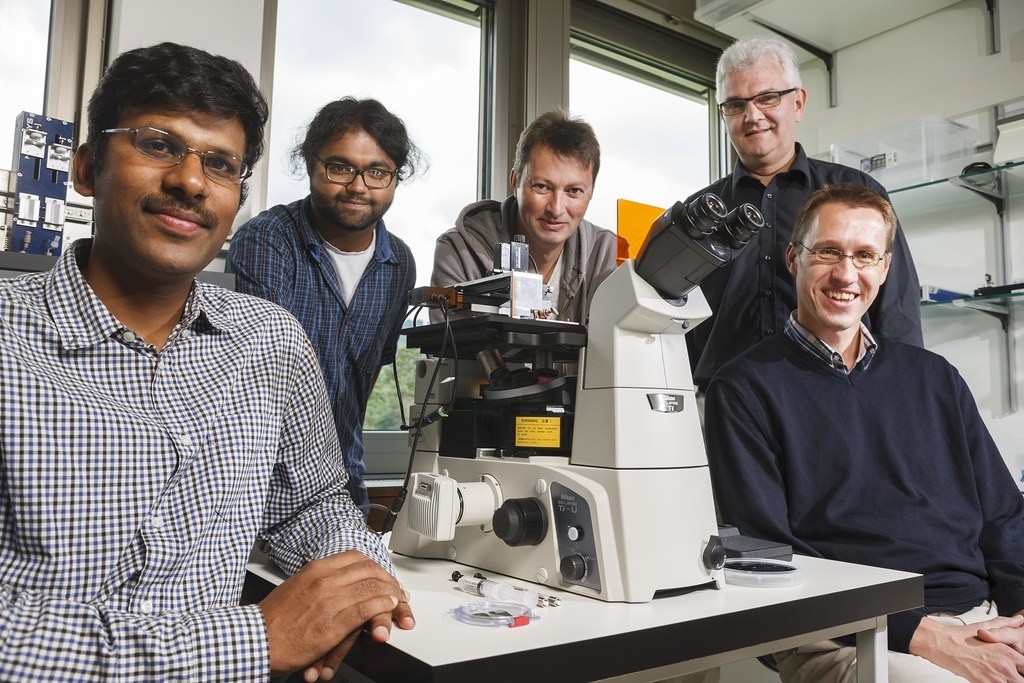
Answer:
724, 557, 799, 588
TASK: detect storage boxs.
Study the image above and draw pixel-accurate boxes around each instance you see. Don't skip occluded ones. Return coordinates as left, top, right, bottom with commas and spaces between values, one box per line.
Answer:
835, 115, 980, 215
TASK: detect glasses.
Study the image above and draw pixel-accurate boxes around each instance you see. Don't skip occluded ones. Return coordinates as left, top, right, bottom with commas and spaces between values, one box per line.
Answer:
101, 127, 252, 185
798, 240, 887, 269
315, 154, 398, 188
718, 87, 796, 116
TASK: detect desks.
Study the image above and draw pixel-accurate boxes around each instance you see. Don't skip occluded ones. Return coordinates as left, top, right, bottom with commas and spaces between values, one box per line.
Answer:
245, 530, 923, 683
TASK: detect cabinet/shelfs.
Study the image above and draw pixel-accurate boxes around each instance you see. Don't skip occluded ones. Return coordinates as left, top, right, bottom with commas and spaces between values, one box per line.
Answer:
884, 161, 1024, 318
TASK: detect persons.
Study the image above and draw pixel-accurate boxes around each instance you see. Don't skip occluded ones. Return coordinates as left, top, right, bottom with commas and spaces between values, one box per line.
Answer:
0, 43, 422, 683
683, 37, 924, 432
705, 184, 1024, 682
428, 106, 617, 377
